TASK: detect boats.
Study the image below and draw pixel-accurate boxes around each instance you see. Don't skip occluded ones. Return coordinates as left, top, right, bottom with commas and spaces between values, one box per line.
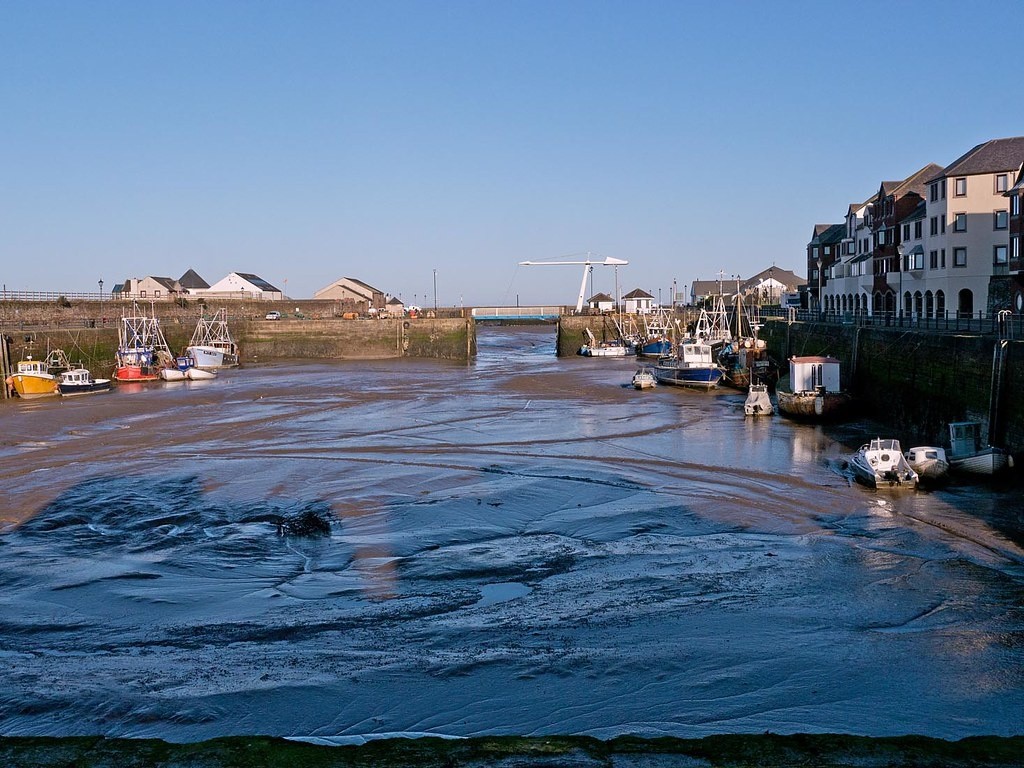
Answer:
47, 349, 75, 379
776, 354, 852, 419
159, 369, 189, 382
944, 420, 1008, 477
108, 299, 175, 382
744, 368, 775, 418
629, 368, 657, 391
849, 437, 919, 490
57, 366, 111, 397
6, 353, 57, 400
178, 304, 242, 367
187, 367, 218, 381
903, 447, 950, 483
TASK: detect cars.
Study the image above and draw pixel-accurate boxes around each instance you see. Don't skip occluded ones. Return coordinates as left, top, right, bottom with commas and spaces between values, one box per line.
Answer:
266, 311, 280, 320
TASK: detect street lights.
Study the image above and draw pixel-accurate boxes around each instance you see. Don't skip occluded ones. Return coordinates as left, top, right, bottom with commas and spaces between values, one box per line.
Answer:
432, 268, 438, 318
768, 267, 775, 305
816, 257, 822, 322
240, 287, 244, 300
97, 278, 105, 328
897, 243, 906, 327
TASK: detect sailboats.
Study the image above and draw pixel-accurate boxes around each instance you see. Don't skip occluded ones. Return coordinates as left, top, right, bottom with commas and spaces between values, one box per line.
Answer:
585, 266, 782, 395
